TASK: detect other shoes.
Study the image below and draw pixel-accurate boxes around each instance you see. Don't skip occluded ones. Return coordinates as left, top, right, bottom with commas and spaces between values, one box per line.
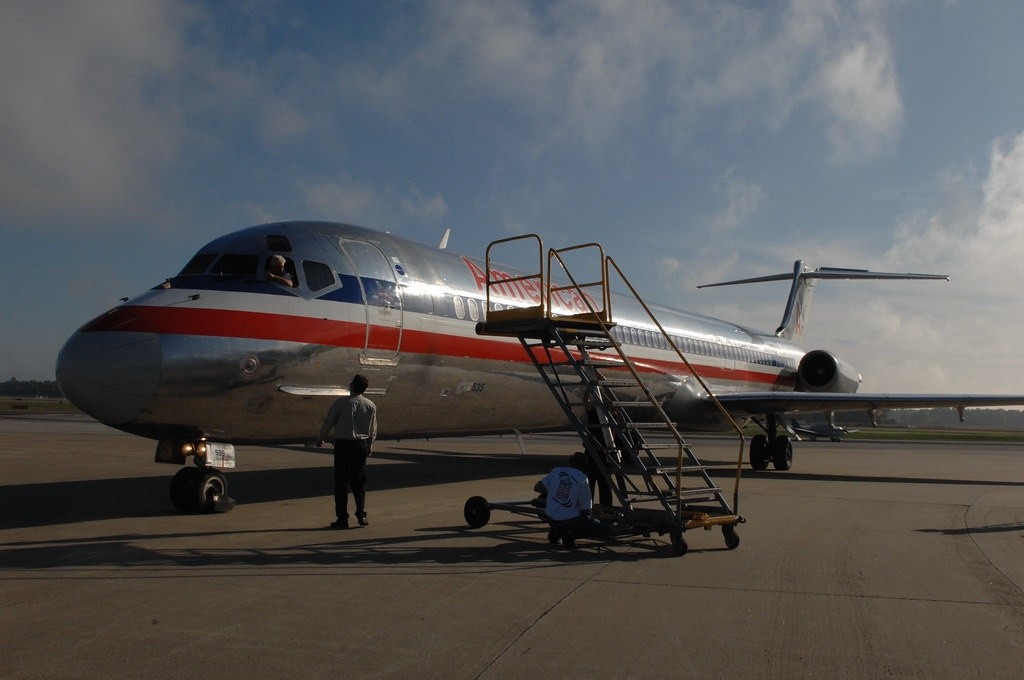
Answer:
548, 527, 575, 547
358, 516, 369, 527
330, 520, 350, 529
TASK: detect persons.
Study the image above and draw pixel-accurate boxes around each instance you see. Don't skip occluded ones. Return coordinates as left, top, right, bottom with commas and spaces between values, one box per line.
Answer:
267, 254, 293, 287
315, 373, 378, 529
534, 452, 593, 548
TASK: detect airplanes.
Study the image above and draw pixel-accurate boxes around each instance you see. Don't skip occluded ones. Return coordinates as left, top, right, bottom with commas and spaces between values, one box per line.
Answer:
789, 412, 860, 442
56, 220, 1024, 515
